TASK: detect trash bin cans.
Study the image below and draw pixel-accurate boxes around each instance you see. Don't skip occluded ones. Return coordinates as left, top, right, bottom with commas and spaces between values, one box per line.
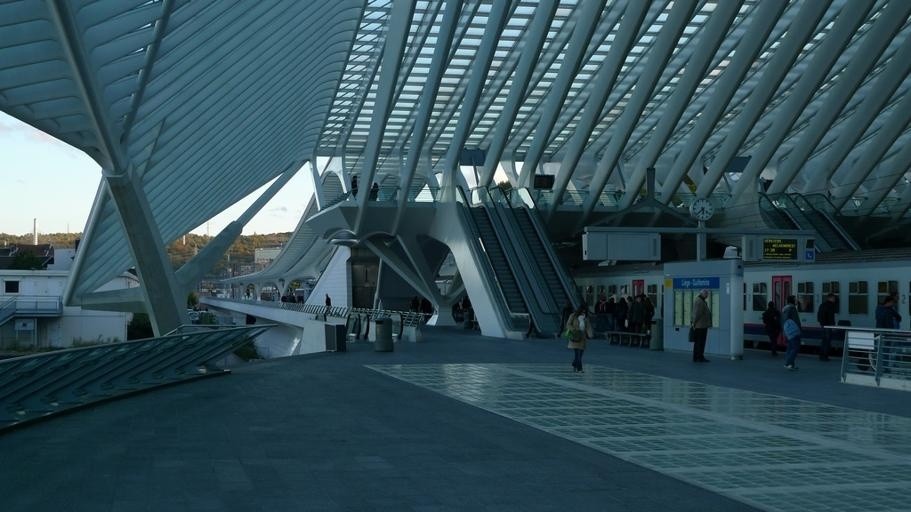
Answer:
375, 318, 393, 352
650, 319, 663, 350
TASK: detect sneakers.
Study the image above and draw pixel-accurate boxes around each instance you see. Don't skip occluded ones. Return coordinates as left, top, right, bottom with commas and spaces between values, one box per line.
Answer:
574, 367, 584, 372
694, 358, 710, 363
784, 363, 800, 371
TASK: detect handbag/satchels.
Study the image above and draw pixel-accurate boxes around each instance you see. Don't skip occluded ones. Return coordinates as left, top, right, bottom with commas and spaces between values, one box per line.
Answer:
783, 318, 801, 340
569, 330, 583, 343
688, 326, 695, 342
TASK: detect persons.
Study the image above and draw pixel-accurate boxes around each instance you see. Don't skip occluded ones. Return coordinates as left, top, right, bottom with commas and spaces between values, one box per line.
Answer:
762, 291, 902, 373
563, 292, 654, 346
409, 295, 474, 330
352, 176, 358, 198
566, 305, 594, 374
370, 183, 379, 201
325, 293, 331, 306
690, 289, 713, 362
247, 289, 304, 304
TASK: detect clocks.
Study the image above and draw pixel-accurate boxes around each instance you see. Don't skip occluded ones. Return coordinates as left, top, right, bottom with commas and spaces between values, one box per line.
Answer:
691, 198, 716, 222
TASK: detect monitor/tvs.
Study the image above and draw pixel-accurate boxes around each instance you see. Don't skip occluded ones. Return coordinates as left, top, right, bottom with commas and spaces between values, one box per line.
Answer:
534, 173, 555, 190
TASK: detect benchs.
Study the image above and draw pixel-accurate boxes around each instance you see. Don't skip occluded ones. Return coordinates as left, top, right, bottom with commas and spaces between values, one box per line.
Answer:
607, 331, 648, 348
